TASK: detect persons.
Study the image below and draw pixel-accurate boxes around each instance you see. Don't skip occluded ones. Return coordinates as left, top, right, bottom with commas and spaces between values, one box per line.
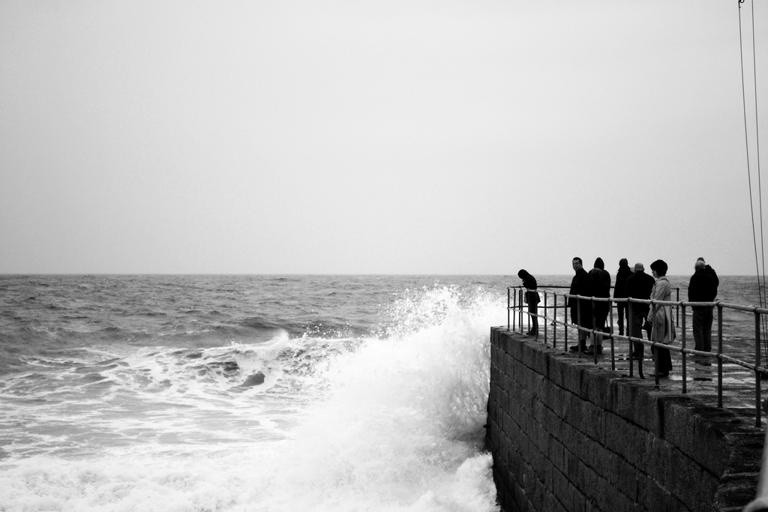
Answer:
688, 258, 719, 357
568, 257, 588, 352
623, 262, 655, 357
646, 260, 676, 377
584, 257, 611, 355
614, 258, 631, 336
518, 269, 540, 335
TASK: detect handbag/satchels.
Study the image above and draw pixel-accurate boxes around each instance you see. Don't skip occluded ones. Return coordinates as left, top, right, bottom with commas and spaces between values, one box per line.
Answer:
642, 319, 652, 331
603, 318, 613, 341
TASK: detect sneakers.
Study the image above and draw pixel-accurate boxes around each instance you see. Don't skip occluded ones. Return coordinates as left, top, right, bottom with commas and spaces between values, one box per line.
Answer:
571, 344, 605, 354
626, 349, 670, 377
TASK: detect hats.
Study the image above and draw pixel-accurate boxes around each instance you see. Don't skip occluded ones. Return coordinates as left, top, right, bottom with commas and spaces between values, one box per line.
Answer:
635, 262, 644, 272
